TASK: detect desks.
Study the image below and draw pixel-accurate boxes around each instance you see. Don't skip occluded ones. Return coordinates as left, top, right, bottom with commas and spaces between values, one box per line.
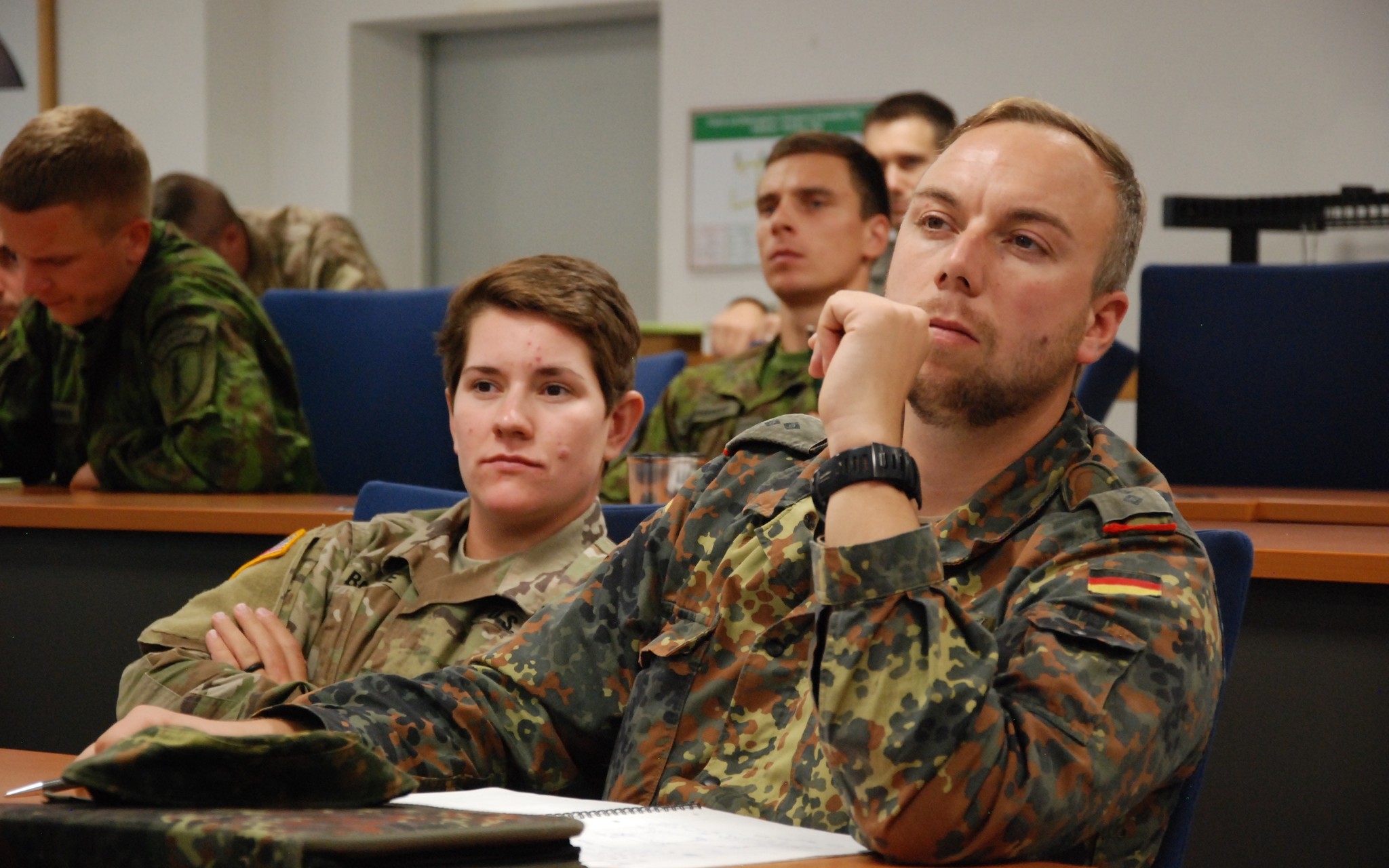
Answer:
3, 477, 1388, 868
0, 746, 1073, 867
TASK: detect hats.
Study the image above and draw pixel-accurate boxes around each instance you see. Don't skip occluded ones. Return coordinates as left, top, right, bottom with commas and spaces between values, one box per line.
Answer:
43, 725, 419, 809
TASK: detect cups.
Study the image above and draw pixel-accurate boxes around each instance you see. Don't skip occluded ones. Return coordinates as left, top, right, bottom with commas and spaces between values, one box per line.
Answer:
626, 451, 708, 506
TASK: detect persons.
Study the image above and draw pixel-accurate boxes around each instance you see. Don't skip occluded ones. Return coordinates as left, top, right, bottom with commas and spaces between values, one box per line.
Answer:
1, 88, 1223, 868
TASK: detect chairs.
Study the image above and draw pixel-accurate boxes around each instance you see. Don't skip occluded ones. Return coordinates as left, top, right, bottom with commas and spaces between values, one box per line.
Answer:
237, 286, 480, 500
1146, 261, 1388, 487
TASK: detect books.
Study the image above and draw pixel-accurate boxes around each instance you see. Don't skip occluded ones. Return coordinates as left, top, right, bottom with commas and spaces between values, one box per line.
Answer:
390, 786, 873, 867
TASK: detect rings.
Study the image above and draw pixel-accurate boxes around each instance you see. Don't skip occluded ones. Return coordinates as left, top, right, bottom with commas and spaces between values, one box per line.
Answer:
244, 661, 265, 673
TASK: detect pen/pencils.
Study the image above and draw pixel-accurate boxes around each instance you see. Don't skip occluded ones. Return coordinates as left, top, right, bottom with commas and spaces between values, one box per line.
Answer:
3, 778, 82, 797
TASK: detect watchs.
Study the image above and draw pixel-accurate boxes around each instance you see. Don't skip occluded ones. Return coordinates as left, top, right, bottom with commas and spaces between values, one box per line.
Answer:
810, 443, 922, 515
